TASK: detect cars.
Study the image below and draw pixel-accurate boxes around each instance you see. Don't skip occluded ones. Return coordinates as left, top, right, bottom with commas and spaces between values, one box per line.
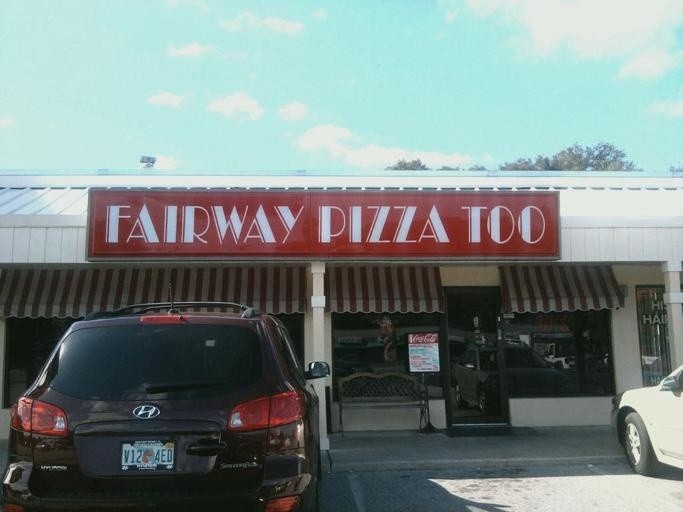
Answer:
448, 339, 568, 414
610, 365, 682, 480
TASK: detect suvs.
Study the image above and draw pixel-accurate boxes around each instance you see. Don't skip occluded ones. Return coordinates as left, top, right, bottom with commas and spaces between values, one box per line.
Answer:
0, 282, 331, 511
